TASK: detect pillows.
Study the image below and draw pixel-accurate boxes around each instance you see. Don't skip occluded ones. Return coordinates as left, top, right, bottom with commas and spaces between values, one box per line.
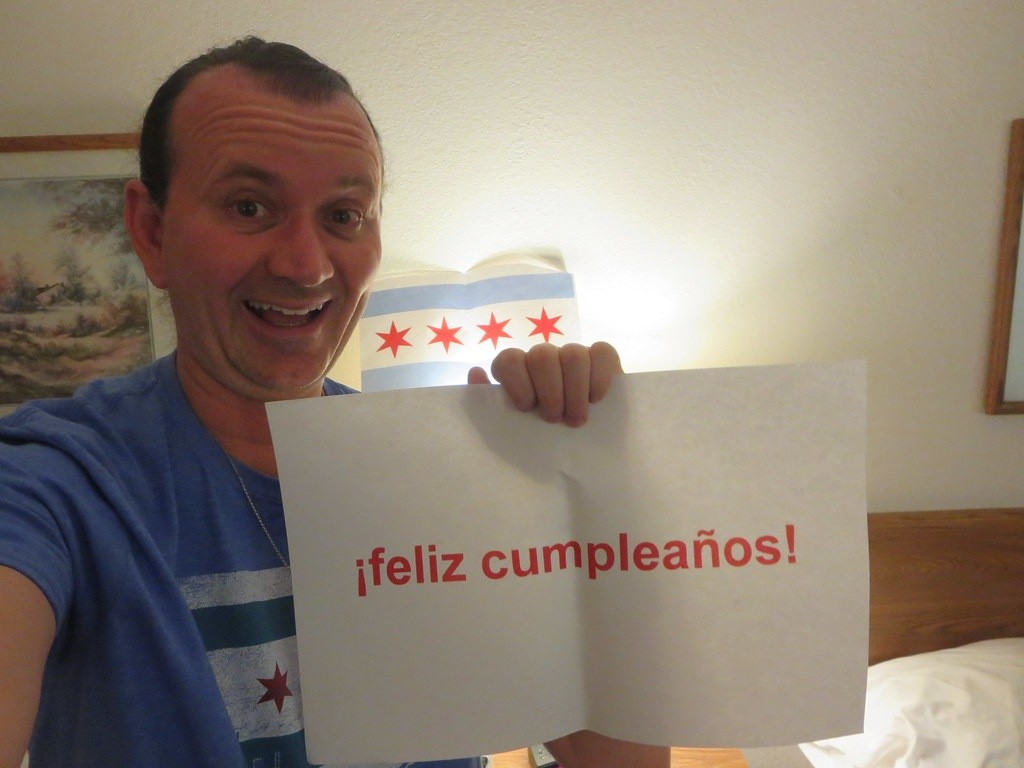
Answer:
799, 639, 1024, 768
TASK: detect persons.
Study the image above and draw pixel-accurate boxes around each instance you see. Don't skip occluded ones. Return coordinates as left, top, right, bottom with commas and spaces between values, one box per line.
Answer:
0, 37, 672, 768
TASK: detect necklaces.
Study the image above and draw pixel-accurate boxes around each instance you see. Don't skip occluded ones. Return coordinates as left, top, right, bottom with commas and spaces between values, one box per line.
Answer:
225, 454, 292, 570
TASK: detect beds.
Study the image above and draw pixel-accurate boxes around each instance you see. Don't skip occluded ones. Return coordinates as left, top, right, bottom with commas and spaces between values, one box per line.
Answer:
741, 504, 1024, 768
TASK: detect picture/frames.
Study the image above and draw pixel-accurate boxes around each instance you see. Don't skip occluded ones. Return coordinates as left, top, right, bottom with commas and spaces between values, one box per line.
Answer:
0, 133, 177, 418
985, 117, 1024, 416
